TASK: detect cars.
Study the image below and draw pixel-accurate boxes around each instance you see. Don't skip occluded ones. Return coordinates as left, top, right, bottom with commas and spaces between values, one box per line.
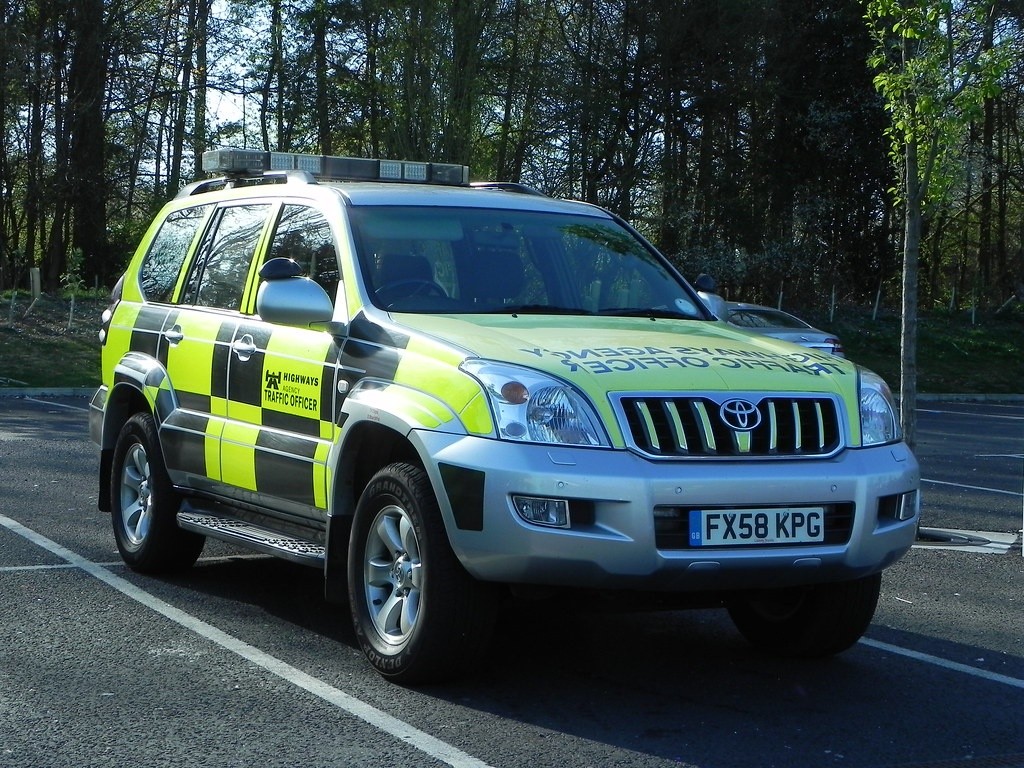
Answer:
722, 301, 845, 359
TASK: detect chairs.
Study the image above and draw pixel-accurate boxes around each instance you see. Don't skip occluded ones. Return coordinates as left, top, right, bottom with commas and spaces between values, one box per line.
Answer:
302, 248, 523, 305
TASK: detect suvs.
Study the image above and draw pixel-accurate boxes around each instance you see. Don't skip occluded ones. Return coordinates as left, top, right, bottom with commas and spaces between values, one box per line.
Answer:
87, 142, 923, 685
